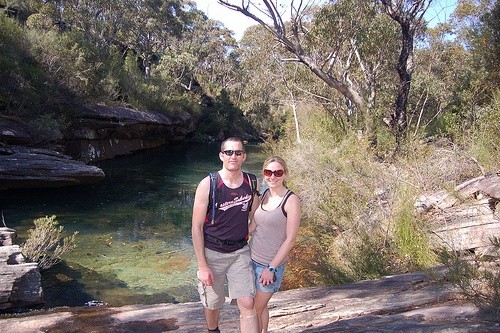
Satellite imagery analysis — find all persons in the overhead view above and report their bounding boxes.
[247,156,300,333]
[192,137,259,333]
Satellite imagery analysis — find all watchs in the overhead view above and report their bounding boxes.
[267,264,278,273]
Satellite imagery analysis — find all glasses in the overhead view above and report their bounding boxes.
[222,150,244,156]
[264,169,286,177]
